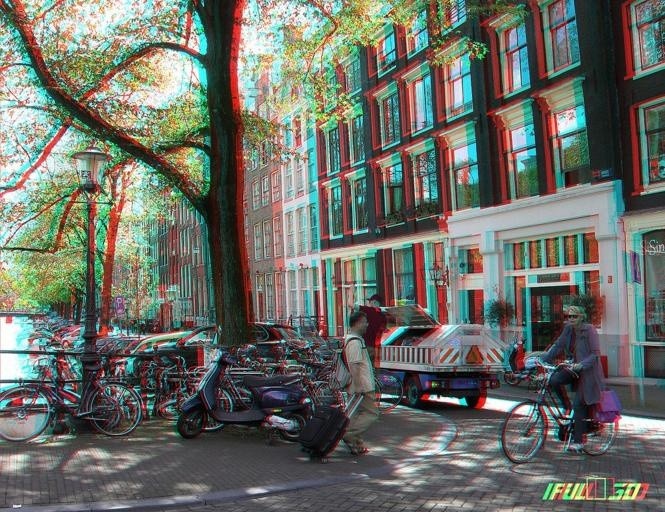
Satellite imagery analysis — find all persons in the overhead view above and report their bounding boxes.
[338,311,381,457]
[520,304,605,453]
[357,294,386,366]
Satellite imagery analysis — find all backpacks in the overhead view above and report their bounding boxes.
[329,336,362,390]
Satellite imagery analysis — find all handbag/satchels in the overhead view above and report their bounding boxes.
[595,390,620,423]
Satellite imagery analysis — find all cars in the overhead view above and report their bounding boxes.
[48,316,309,391]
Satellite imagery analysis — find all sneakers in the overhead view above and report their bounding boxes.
[563,407,574,419]
[570,442,584,450]
[344,437,366,457]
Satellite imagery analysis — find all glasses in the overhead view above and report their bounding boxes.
[567,314,583,319]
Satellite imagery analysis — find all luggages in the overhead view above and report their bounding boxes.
[298,392,365,463]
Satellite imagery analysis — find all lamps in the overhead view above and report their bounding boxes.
[430,262,450,287]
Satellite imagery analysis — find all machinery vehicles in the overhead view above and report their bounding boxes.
[375,304,511,408]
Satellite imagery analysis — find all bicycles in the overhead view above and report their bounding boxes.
[0,354,145,442]
[501,356,618,464]
[157,343,403,441]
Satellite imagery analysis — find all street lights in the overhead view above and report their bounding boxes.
[71,146,111,433]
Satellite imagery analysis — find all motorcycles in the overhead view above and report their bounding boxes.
[504,339,544,385]
